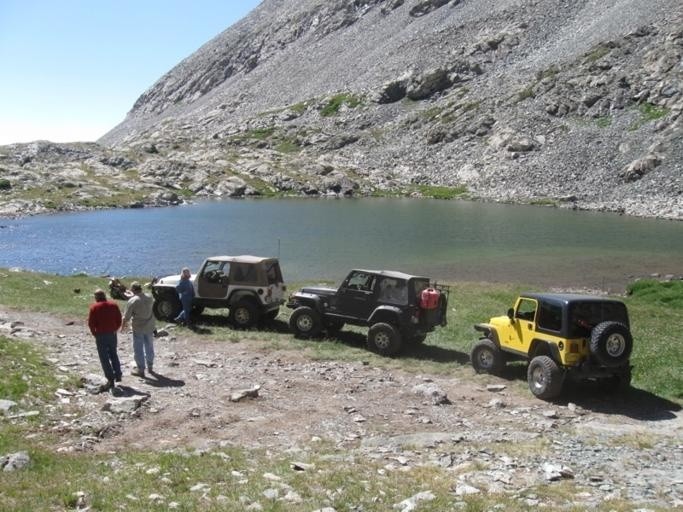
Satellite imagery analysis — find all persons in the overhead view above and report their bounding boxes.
[173,267,198,331]
[108,276,159,301]
[120,280,155,377]
[88,289,123,391]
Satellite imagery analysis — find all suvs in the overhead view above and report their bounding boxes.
[146,254,288,330]
[284,266,453,357]
[467,290,637,404]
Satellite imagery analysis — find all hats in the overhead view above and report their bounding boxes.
[94,288,106,300]
[128,280,141,292]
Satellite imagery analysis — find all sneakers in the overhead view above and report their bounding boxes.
[107,318,188,382]
